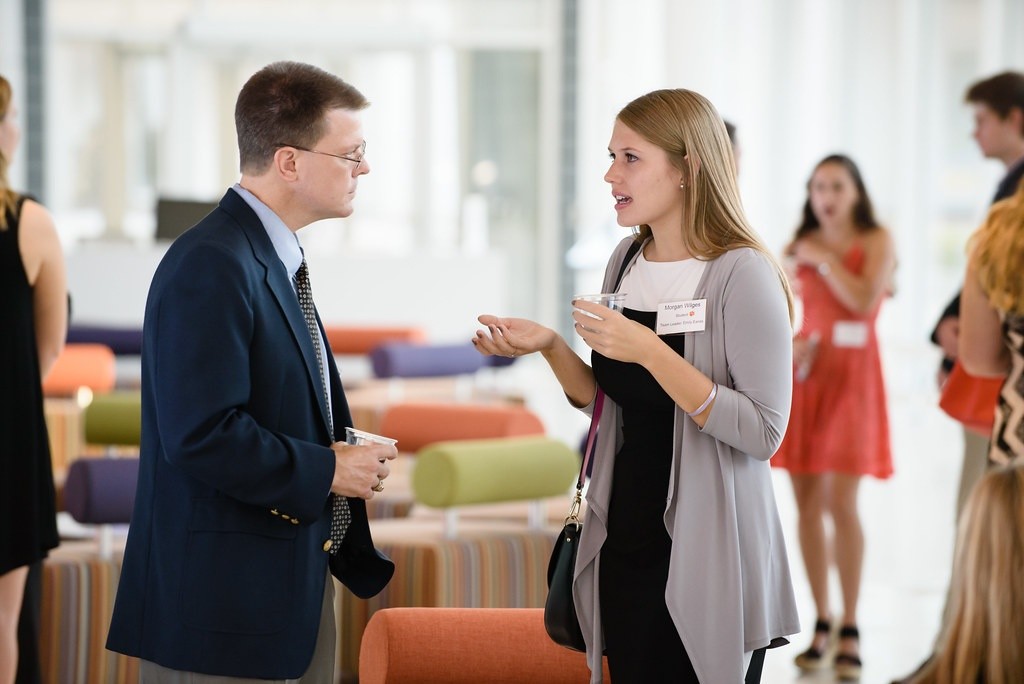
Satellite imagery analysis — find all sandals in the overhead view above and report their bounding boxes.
[796,619,837,670]
[836,625,861,679]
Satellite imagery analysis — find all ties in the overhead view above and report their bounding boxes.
[295,247,352,555]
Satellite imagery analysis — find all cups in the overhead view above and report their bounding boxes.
[570,292,627,333]
[345,426,399,465]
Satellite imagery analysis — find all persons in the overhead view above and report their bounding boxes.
[105,60,398,684]
[472,89,800,684]
[771,154,897,679]
[891,71,1024,684]
[0,74,67,684]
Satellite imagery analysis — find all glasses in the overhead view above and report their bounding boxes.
[270,139,366,169]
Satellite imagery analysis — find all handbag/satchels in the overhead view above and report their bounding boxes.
[541,522,607,657]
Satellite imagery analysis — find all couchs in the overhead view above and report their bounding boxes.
[314,325,610,684]
[37,322,170,684]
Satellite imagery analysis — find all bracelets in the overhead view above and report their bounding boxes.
[687,383,716,417]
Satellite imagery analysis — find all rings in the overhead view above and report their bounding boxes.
[509,348,517,356]
[372,480,384,492]
